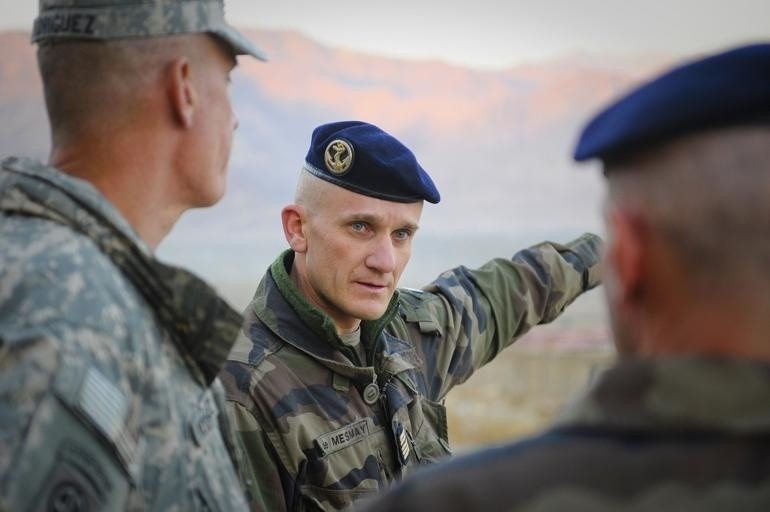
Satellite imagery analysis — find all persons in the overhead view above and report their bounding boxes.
[218,120,607,511]
[349,43,769,511]
[1,0,279,511]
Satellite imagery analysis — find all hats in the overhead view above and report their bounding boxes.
[569,39,770,176]
[29,0,270,65]
[302,119,442,207]
